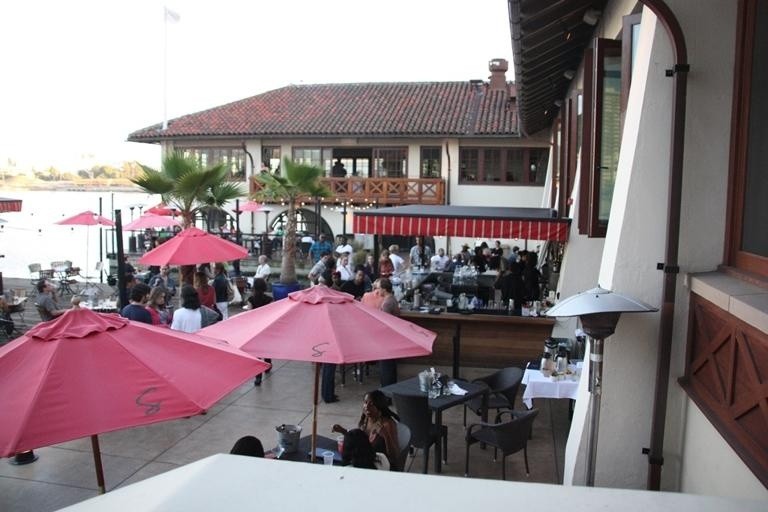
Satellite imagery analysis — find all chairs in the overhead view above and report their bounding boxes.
[139,232,312,265]
[464,367,539,480]
[392,392,448,473]
[0,260,80,342]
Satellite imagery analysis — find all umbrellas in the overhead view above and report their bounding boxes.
[0,296,271,492]
[232,202,267,236]
[189,283,438,461]
[144,202,183,218]
[56,211,115,281]
[122,213,180,252]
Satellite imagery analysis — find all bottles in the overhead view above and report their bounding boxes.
[281,424,285,432]
[412,289,515,312]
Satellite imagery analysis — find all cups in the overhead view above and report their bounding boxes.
[336,435,344,452]
[323,451,334,466]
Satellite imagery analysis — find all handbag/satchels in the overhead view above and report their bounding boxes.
[210,306,223,321]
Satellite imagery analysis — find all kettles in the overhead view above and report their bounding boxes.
[541,337,568,376]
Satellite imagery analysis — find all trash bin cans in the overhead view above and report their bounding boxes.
[271,282,301,300]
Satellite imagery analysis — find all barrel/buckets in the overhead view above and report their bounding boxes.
[419,371,441,391]
[3,288,16,303]
[274,424,303,455]
[273,281,304,300]
[230,278,247,293]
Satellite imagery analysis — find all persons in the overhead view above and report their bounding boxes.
[428,241,503,273]
[125,262,176,325]
[230,436,266,457]
[143,225,180,250]
[0,295,17,336]
[358,390,401,456]
[410,237,431,268]
[495,247,540,314]
[332,158,407,207]
[248,255,272,385]
[38,277,70,320]
[330,426,393,471]
[322,286,339,403]
[180,261,230,321]
[300,231,405,298]
[375,278,400,386]
[256,225,276,247]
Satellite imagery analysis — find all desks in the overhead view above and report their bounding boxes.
[265,434,350,465]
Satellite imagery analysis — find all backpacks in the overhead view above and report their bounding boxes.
[222,272,234,302]
